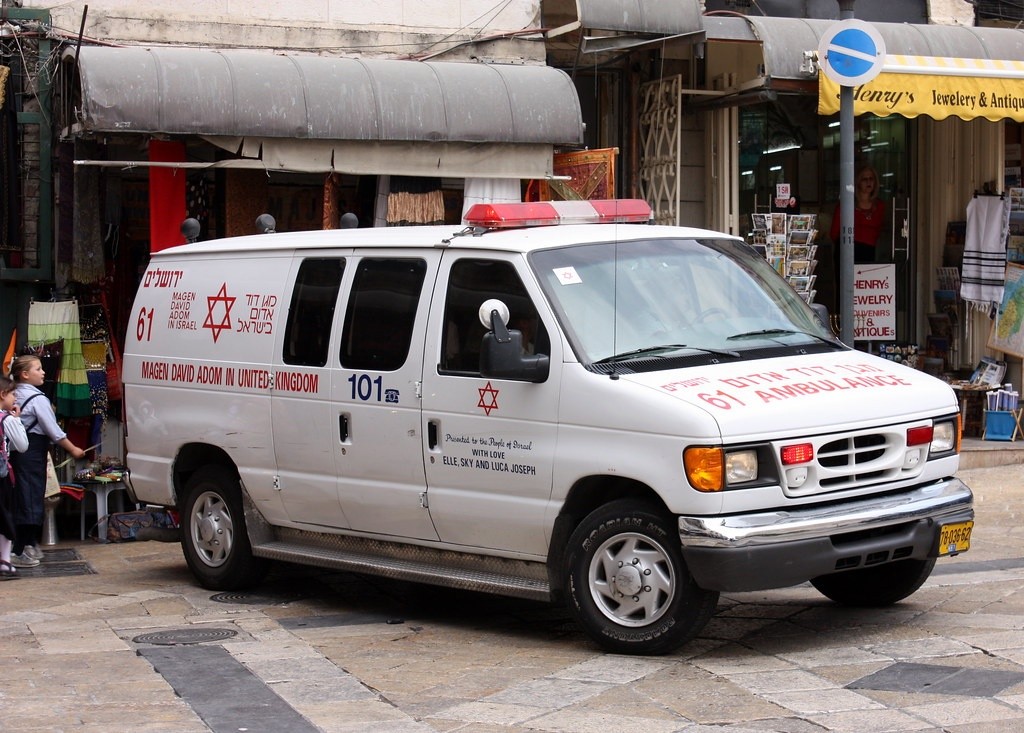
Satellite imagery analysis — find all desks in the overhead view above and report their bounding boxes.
[949,380,1001,432]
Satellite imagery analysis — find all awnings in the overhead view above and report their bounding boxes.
[804,50,1024,124]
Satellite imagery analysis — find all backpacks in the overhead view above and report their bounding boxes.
[0,413,17,488]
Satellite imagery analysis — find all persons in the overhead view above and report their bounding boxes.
[0,356,85,580]
[830,164,888,263]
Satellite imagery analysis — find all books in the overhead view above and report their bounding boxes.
[749,213,817,307]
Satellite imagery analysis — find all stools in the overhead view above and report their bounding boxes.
[81,482,141,542]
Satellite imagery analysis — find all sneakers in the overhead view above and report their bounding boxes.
[23,546,44,560]
[10,553,40,567]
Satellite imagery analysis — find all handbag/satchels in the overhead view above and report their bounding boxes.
[89,508,180,544]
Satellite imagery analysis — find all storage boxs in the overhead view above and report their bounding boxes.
[980,408,1024,442]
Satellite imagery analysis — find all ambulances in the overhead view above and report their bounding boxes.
[121,199,974,655]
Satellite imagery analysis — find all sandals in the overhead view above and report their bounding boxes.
[0,560,21,580]
